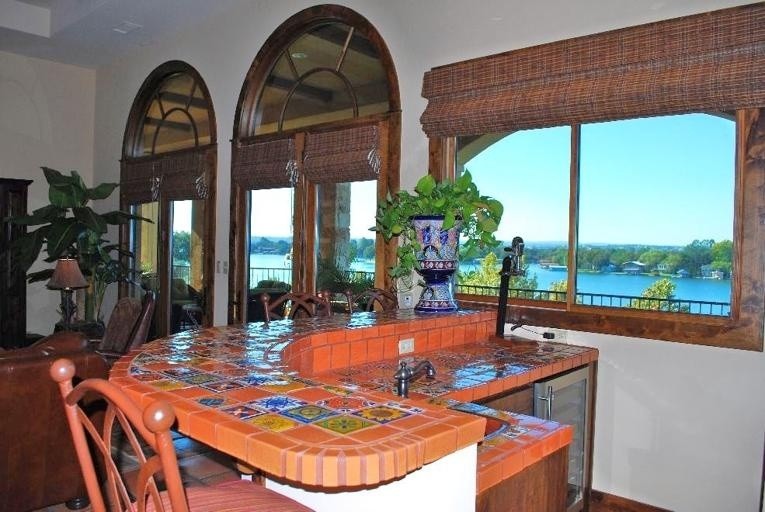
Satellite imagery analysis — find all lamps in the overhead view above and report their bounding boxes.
[45,258,90,329]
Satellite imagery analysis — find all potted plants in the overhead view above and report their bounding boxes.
[368,169,505,315]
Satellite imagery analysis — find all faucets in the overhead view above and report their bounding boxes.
[502,236,527,278]
[395,358,437,398]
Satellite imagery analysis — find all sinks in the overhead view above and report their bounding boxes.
[448,405,508,443]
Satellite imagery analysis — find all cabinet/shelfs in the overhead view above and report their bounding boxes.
[534,363,597,511]
